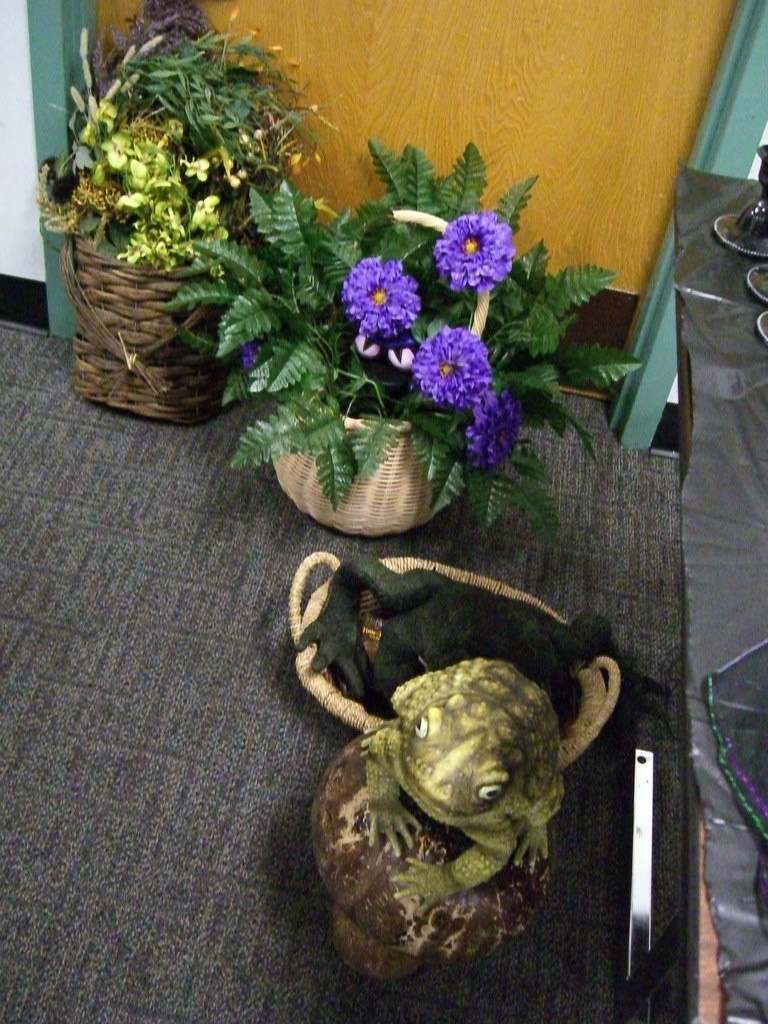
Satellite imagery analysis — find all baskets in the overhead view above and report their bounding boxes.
[287,553,624,774]
[266,213,491,540]
[61,212,228,425]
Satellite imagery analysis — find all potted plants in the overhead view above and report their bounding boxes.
[54,207,230,425]
[255,382,493,539]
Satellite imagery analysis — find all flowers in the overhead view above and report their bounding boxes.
[39,5,299,278]
[178,140,633,532]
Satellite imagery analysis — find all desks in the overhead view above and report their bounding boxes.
[663,163,768,1019]
[0,316,695,1015]
